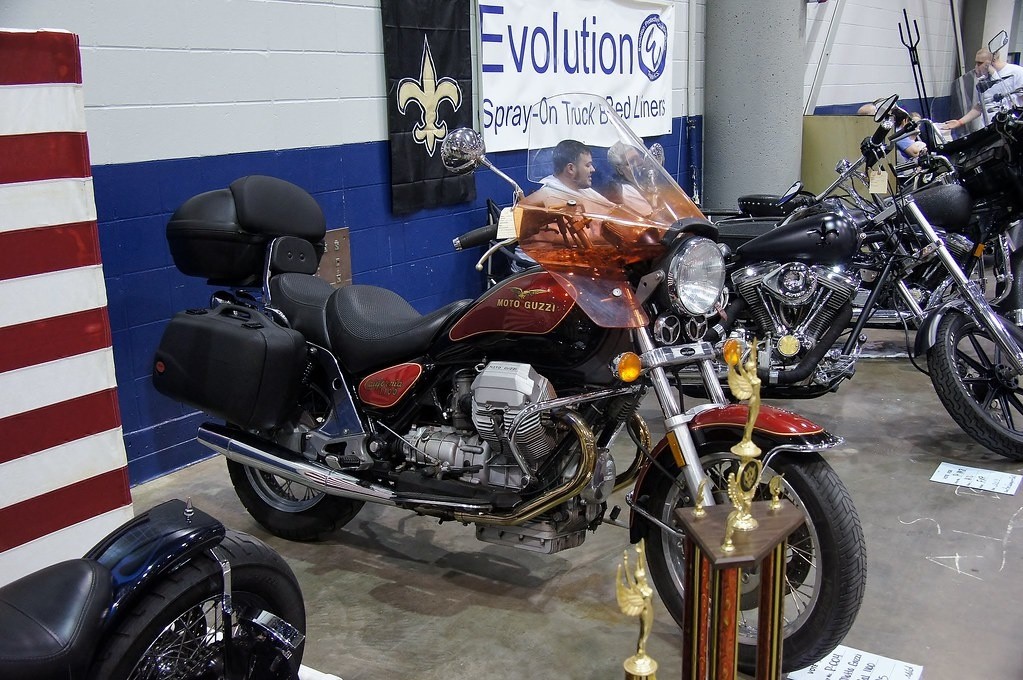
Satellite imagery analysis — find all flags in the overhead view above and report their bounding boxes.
[380,0,476,215]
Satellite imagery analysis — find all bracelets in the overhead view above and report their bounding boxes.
[958,120,963,127]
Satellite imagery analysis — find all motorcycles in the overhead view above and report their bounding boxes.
[487,123,1023,461]
[697,29,1023,325]
[0,499,305,680]
[153,93,866,675]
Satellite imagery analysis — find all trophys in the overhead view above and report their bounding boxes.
[617,335,804,680]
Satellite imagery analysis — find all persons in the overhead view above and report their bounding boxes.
[511,139,614,274]
[607,141,645,204]
[858,104,928,174]
[941,47,1023,131]
